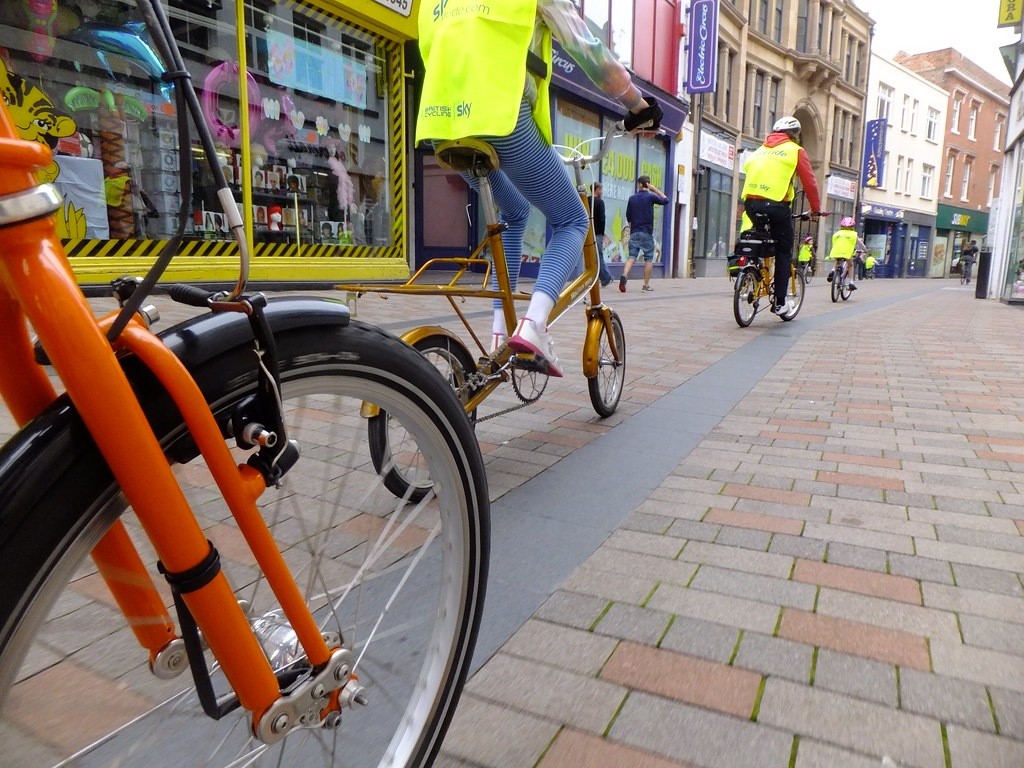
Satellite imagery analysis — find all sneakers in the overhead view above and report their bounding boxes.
[508,318,565,377]
[771,300,796,315]
[489,333,508,353]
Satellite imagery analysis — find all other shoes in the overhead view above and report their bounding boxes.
[849,283,857,290]
[601,276,615,288]
[640,285,654,293]
[827,270,835,282]
[619,275,627,292]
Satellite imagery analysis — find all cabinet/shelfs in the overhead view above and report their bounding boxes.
[228,188,315,247]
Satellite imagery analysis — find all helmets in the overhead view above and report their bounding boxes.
[773,116,801,131]
[971,240,977,243]
[805,236,813,242]
[840,217,855,227]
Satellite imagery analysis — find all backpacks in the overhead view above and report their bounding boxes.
[963,242,973,253]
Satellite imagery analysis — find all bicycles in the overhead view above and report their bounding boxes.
[332,121,662,504]
[734,211,852,328]
[960,259,977,285]
[1,0,493,768]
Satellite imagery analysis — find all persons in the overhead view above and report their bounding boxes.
[269,173,279,189]
[711,237,726,257]
[298,211,305,225]
[619,175,670,293]
[321,223,336,240]
[797,236,818,275]
[286,176,301,193]
[958,240,979,283]
[256,207,265,223]
[741,116,821,317]
[267,205,283,232]
[414,0,663,377]
[255,171,263,188]
[827,216,879,291]
[739,210,753,233]
[585,182,616,287]
[320,135,354,209]
[214,214,224,232]
[205,213,214,231]
[336,223,343,238]
